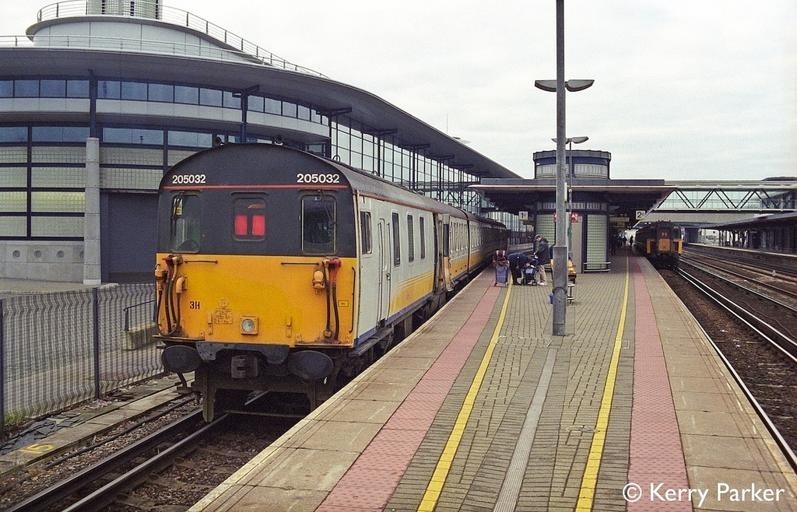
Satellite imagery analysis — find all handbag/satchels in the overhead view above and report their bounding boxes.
[493,249,509,265]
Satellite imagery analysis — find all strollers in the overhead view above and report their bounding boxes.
[522,250,537,287]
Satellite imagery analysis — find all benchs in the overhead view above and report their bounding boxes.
[548,258,575,305]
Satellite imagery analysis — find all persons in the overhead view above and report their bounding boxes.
[508,253,532,285]
[609,234,633,256]
[534,233,550,285]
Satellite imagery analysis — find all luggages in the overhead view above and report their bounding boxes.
[494,264,509,288]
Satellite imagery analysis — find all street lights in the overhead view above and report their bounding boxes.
[549,135,590,259]
[535,76,594,338]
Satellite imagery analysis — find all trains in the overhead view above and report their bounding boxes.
[632,219,682,266]
[151,139,513,424]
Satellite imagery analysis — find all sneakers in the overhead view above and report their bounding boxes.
[537,281,548,286]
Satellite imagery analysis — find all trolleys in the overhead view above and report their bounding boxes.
[491,249,509,288]
